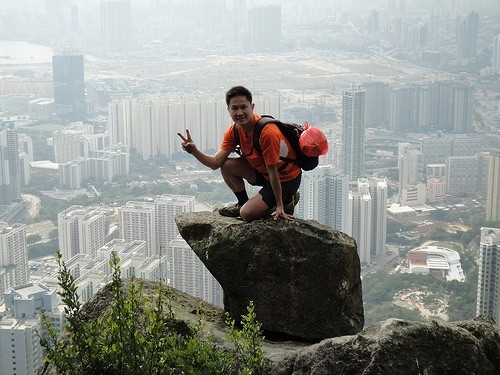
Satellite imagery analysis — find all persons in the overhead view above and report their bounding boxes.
[177,85,302,222]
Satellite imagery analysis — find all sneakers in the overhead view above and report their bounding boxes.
[218,201,245,218]
[283,191,301,218]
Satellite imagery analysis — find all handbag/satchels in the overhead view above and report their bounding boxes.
[298,120,329,157]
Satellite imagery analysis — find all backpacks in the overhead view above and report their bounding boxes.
[232,113,319,171]
